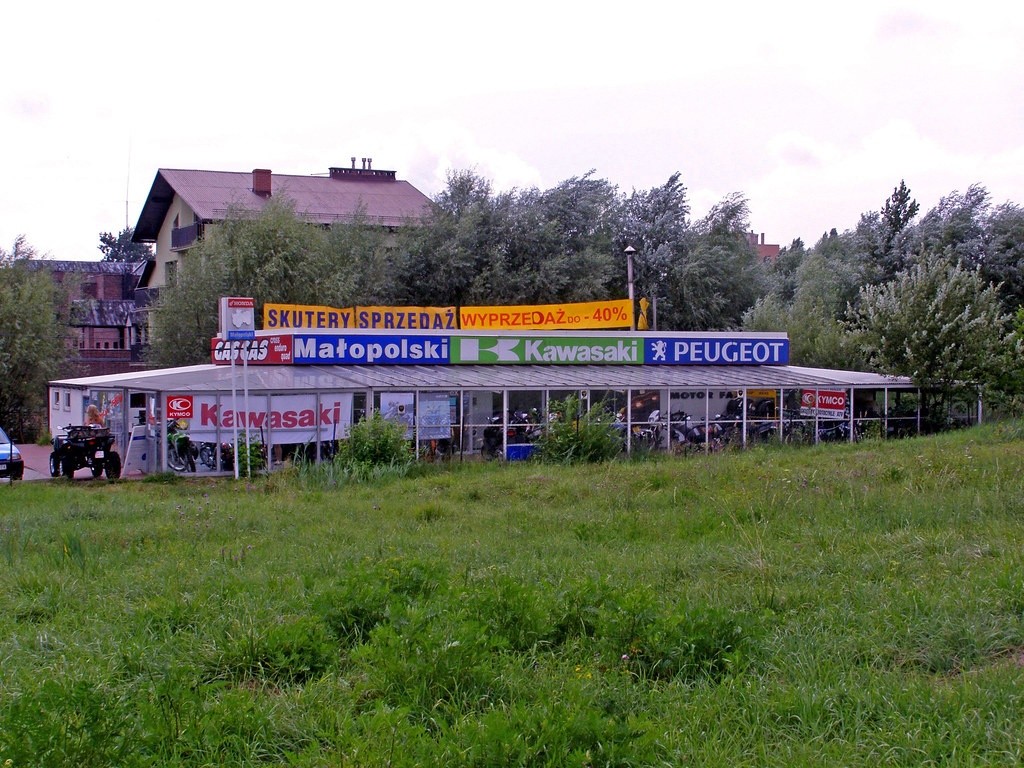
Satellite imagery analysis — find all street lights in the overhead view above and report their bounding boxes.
[623,245,637,331]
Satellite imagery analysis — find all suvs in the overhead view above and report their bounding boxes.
[49,422,122,484]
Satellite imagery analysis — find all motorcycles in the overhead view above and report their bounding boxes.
[155,415,200,473]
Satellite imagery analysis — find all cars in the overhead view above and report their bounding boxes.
[0,426,24,480]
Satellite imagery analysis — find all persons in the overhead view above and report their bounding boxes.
[83,404,105,479]
[274,443,306,464]
[435,427,458,463]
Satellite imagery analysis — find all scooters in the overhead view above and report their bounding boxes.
[199,397,900,476]
